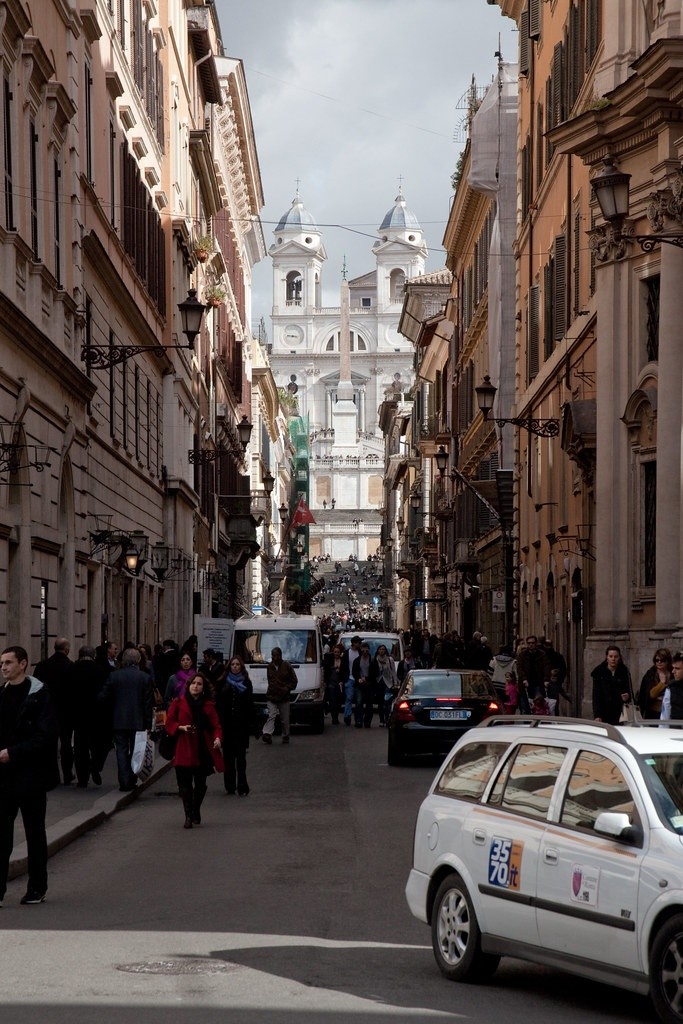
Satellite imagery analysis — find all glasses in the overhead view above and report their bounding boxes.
[361,649,369,653]
[655,658,667,662]
[527,641,535,644]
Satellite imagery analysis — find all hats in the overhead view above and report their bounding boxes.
[404,648,412,652]
[351,636,365,643]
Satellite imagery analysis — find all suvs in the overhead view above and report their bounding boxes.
[406,715,683,1024]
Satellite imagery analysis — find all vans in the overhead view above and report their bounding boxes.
[335,629,405,707]
[230,614,324,732]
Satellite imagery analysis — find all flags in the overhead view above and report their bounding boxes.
[291,499,316,526]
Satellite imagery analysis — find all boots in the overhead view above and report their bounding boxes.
[192,784,208,825]
[179,784,193,829]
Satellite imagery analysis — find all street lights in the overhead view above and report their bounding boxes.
[475,375,560,440]
[410,489,453,522]
[281,542,304,560]
[81,288,206,369]
[396,516,414,540]
[434,446,461,482]
[386,534,399,552]
[590,153,683,253]
[264,503,288,527]
[188,414,254,465]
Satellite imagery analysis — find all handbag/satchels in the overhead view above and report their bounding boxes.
[383,686,399,705]
[618,703,642,722]
[131,728,156,782]
[158,725,181,761]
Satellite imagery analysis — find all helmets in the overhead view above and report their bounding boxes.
[472,631,483,641]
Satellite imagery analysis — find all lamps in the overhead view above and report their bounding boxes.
[386,534,393,550]
[434,446,480,481]
[187,414,253,465]
[311,566,316,576]
[280,542,302,559]
[589,152,683,252]
[218,471,275,498]
[409,491,437,515]
[79,288,207,370]
[91,528,137,575]
[120,530,149,576]
[396,517,414,537]
[142,541,171,583]
[474,376,560,438]
[261,503,289,527]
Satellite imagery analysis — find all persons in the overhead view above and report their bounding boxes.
[214,656,254,797]
[261,647,298,744]
[398,624,493,691]
[312,547,384,654]
[489,636,573,716]
[332,498,336,509]
[639,648,683,728]
[324,636,397,728]
[323,500,327,508]
[309,427,380,465]
[33,638,154,792]
[0,646,48,904]
[164,672,222,829]
[590,645,634,725]
[139,634,226,798]
[353,518,363,526]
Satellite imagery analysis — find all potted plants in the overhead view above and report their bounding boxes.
[209,288,227,307]
[195,236,211,261]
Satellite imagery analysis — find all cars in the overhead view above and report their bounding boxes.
[388,669,508,767]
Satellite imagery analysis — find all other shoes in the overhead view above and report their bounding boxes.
[63,774,76,785]
[329,715,391,731]
[75,780,87,789]
[262,733,273,745]
[282,736,290,746]
[88,763,102,785]
[19,884,49,904]
